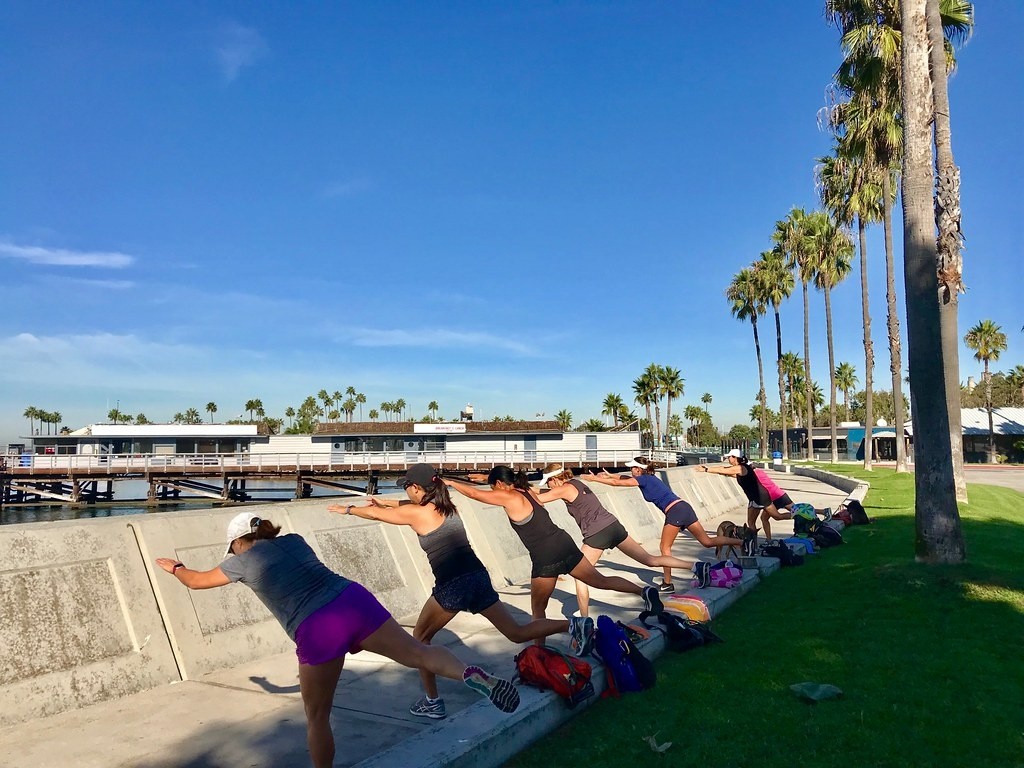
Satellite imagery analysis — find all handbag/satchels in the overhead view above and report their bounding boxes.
[789,502,845,547]
[832,499,869,527]
[759,538,804,570]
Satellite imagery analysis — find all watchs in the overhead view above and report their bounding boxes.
[347,505,356,515]
[173,563,185,575]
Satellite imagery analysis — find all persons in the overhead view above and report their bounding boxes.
[536,463,711,618]
[156,512,520,768]
[327,462,594,719]
[694,449,832,556]
[580,456,755,593]
[441,465,664,646]
[242,446,248,457]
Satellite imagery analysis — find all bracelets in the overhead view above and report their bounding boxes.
[705,468,708,472]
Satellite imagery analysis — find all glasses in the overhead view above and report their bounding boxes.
[402,482,412,491]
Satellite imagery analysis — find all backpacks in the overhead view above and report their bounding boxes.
[640,609,723,654]
[509,643,596,709]
[689,559,744,589]
[591,614,658,700]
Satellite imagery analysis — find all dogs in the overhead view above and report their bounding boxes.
[715,521,762,563]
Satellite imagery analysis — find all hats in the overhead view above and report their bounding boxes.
[222,512,264,560]
[396,463,437,486]
[724,448,744,458]
[625,459,648,469]
[538,467,564,486]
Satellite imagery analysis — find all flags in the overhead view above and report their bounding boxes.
[461,411,467,418]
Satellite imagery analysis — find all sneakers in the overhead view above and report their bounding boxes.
[568,615,594,657]
[824,507,832,522]
[642,585,664,613]
[692,560,711,589]
[742,539,755,557]
[655,578,675,594]
[409,695,447,719]
[462,665,520,714]
